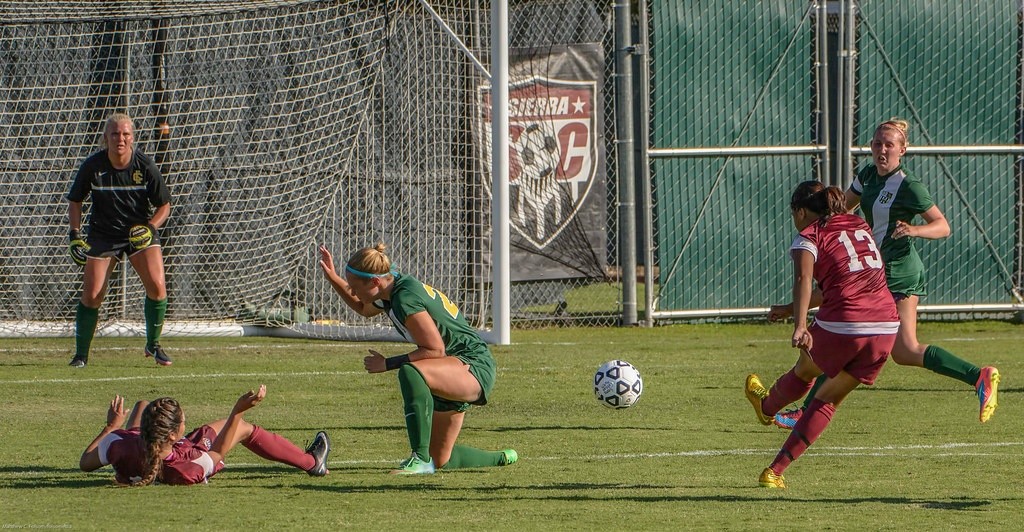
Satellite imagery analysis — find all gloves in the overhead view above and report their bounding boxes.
[130,224,156,251]
[69,228,91,265]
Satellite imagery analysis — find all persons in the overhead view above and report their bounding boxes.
[316,243,519,476]
[744,178,901,490]
[67,114,174,369]
[79,381,330,487]
[773,120,999,432]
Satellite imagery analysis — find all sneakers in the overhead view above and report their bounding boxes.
[69,355,88,367]
[145,341,171,365]
[488,449,518,464]
[975,366,999,423]
[760,467,786,489]
[305,431,330,476]
[774,409,805,430]
[390,453,435,474]
[745,373,774,427]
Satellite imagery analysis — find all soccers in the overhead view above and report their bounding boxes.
[590,357,646,413]
